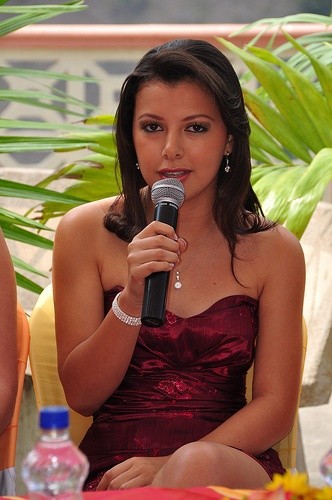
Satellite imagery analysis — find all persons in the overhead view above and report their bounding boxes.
[0,223,19,434]
[51,38,307,492]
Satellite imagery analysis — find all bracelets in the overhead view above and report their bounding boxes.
[112,292,142,327]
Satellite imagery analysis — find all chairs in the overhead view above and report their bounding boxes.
[31,282,311,485]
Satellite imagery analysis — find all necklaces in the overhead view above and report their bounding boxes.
[147,214,213,289]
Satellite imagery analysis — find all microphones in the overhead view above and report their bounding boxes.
[141,178,185,326]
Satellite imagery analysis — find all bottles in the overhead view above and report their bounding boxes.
[20,407,90,500]
